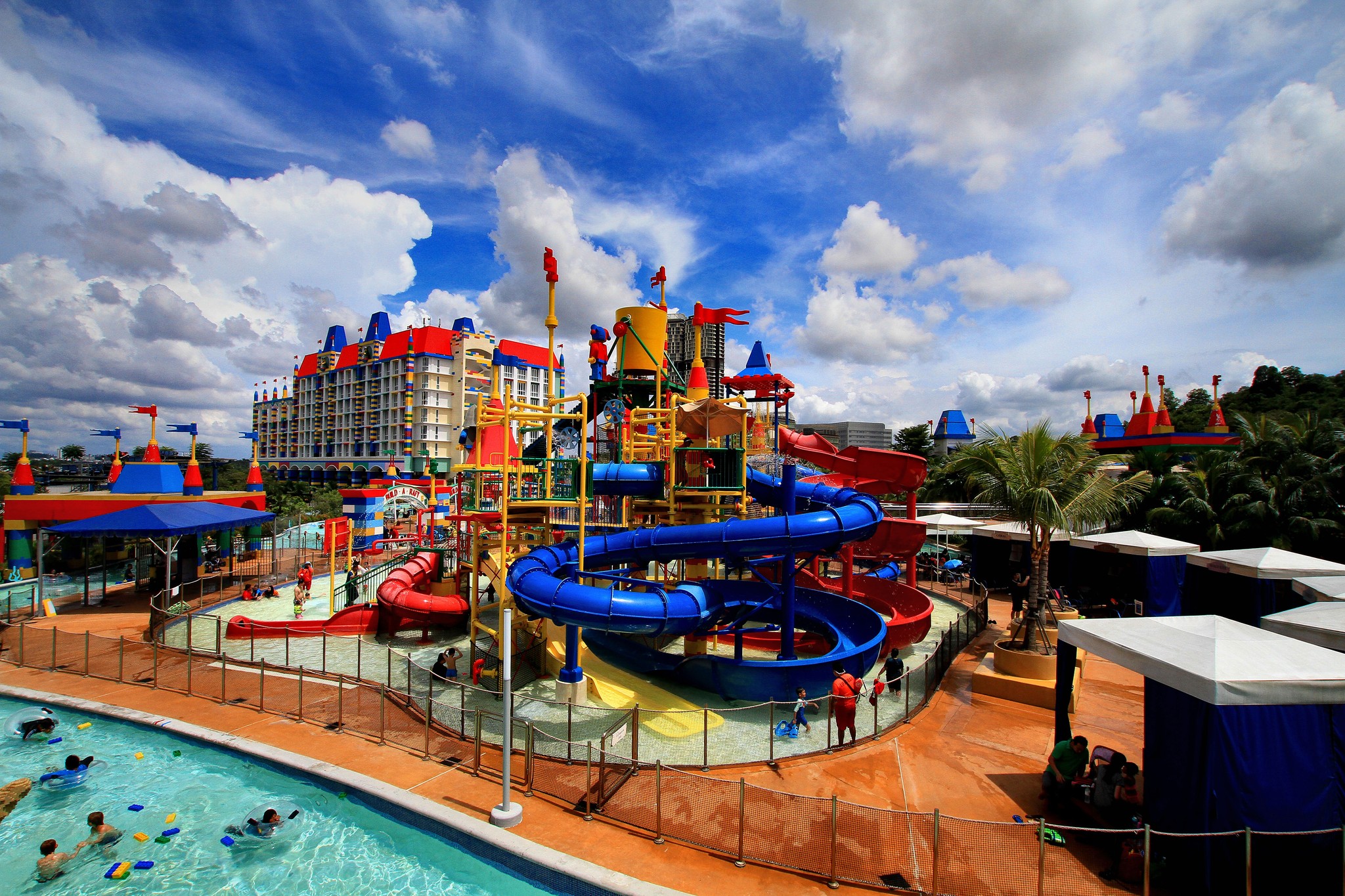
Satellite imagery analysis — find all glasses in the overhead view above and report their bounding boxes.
[685,439,692,442]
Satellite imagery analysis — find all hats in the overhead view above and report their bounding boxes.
[590,324,611,342]
[683,437,694,444]
[833,661,845,675]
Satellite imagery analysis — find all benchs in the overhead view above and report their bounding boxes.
[1072,793,1115,829]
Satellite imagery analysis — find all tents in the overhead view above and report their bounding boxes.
[912,514,987,583]
[1054,614,1345,896]
[968,516,1344,654]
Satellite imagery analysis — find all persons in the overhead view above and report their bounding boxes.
[1121,762,1140,805]
[13,717,55,741]
[877,648,904,704]
[33,839,83,883]
[1093,752,1129,830]
[443,648,463,685]
[75,812,124,851]
[792,687,819,739]
[830,662,857,751]
[432,653,458,682]
[567,368,770,564]
[225,808,284,841]
[916,549,1031,629]
[40,459,551,619]
[30,754,94,791]
[1042,735,1089,811]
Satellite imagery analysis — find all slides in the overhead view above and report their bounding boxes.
[224,422,935,740]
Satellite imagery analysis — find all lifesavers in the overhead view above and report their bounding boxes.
[2,706,60,739]
[241,800,305,842]
[37,574,72,585]
[43,585,79,595]
[42,760,109,791]
[76,575,97,582]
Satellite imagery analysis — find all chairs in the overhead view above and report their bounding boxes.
[1054,588,1089,612]
